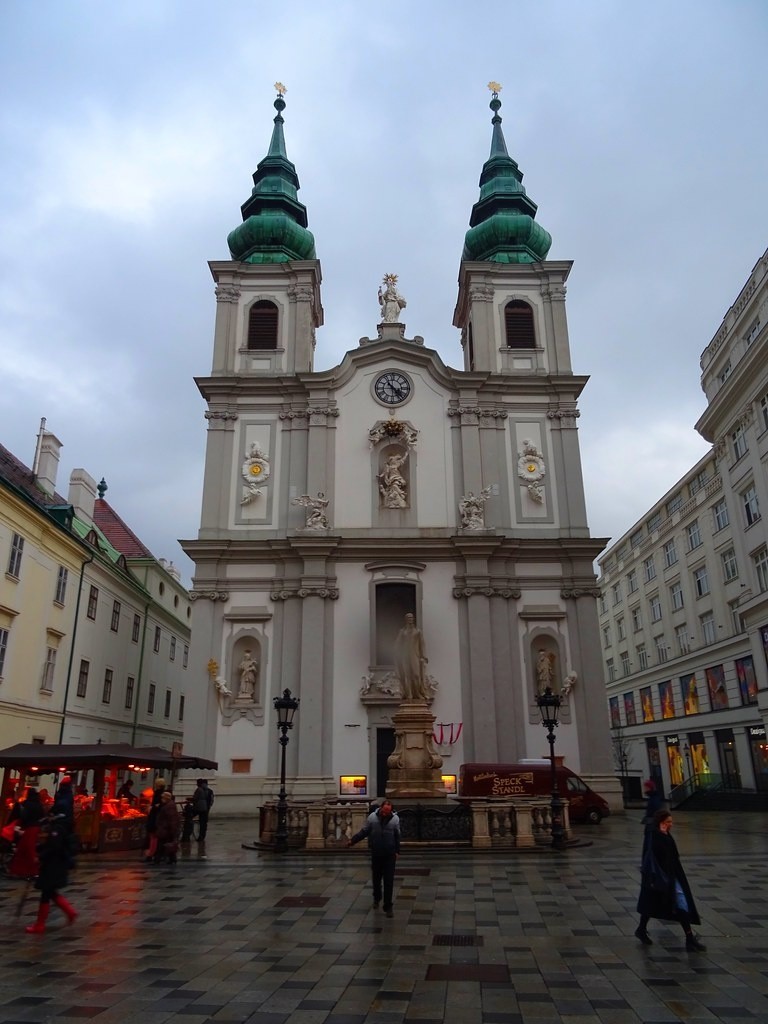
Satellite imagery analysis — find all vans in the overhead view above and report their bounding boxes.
[458,759,610,826]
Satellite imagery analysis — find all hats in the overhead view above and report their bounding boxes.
[655,811,671,825]
[161,792,172,800]
[645,780,655,789]
[155,778,165,786]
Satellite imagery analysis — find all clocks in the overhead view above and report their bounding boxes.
[375,373,410,404]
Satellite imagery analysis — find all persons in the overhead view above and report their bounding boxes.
[359,670,378,695]
[537,649,554,696]
[0,775,214,934]
[378,273,407,322]
[237,648,257,697]
[640,780,665,862]
[345,800,402,917]
[392,613,429,700]
[561,671,577,695]
[634,808,709,951]
[215,677,232,696]
[239,450,544,531]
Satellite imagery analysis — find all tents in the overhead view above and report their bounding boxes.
[0,743,218,801]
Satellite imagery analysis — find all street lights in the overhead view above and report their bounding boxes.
[536,686,567,849]
[271,688,298,854]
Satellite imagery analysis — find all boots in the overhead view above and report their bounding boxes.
[24,903,49,934]
[52,893,79,926]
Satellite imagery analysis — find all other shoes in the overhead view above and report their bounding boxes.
[686,935,708,953]
[387,908,394,918]
[373,901,379,910]
[634,926,652,946]
